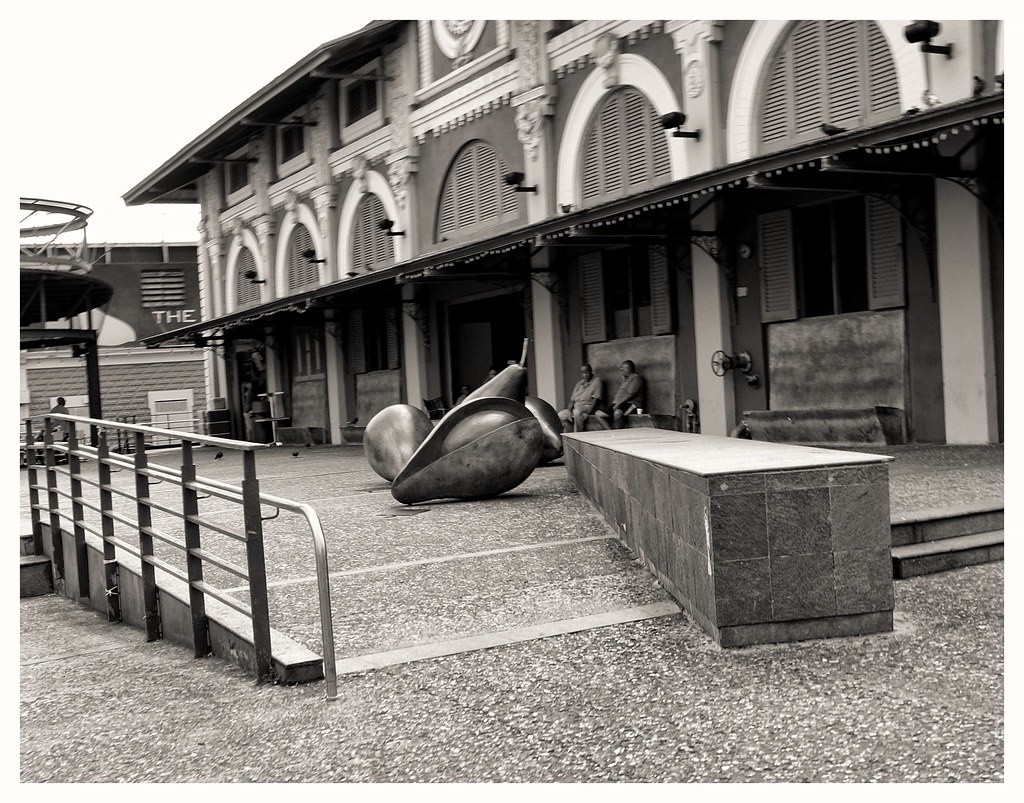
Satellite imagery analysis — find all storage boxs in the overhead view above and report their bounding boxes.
[197,398,231,446]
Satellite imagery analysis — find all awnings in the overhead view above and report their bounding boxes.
[138,89,1004,349]
[122,20,410,204]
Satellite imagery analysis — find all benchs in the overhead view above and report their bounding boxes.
[584,413,677,431]
[742,405,905,447]
[340,426,368,446]
[277,427,327,445]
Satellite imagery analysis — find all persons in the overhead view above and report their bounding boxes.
[594,360,645,431]
[558,362,602,433]
[453,358,518,405]
[36,395,71,463]
[243,381,267,441]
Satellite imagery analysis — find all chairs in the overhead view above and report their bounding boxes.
[422,396,450,421]
